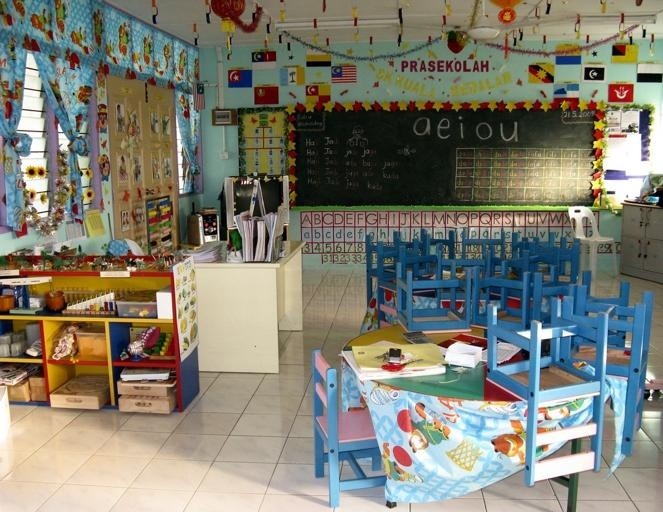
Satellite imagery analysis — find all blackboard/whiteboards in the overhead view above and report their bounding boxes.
[284,100,607,211]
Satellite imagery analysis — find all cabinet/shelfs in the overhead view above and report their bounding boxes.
[187,209,220,246]
[621,202,663,284]
[0,255,199,412]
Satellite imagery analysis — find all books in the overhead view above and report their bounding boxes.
[340,339,446,381]
[119,368,169,381]
[193,211,279,262]
[83,209,107,238]
[353,344,446,372]
[0,362,39,386]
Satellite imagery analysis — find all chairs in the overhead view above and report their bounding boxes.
[359,228,630,348]
[312,349,387,508]
[484,298,603,488]
[564,285,654,457]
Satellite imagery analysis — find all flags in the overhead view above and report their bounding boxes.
[225,48,359,105]
[528,40,662,104]
[192,82,206,111]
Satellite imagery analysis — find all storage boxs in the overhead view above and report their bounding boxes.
[74,325,107,357]
[115,289,159,318]
[8,376,48,403]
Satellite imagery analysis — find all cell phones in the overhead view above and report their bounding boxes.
[388,348,401,364]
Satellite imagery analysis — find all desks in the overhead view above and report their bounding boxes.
[195,237,306,373]
[343,316,632,511]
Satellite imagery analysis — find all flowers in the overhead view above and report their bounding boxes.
[23,143,95,237]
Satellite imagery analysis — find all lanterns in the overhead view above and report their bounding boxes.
[209,1,245,34]
[488,0,523,10]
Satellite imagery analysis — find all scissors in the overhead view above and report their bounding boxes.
[381,359,422,371]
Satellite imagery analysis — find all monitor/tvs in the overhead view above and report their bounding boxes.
[218,174,289,229]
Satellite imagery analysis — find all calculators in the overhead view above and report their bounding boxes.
[401,331,435,344]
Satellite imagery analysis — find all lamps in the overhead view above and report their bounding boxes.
[576,14,656,25]
[467,27,500,42]
[275,18,404,35]
